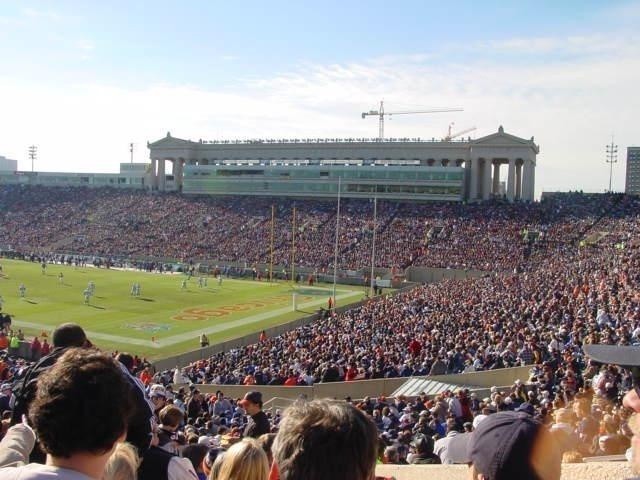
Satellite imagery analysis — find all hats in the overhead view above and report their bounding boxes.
[464,411,560,480]
[583,343,640,366]
[53,323,92,348]
[149,384,167,396]
[239,392,262,405]
[410,437,434,452]
